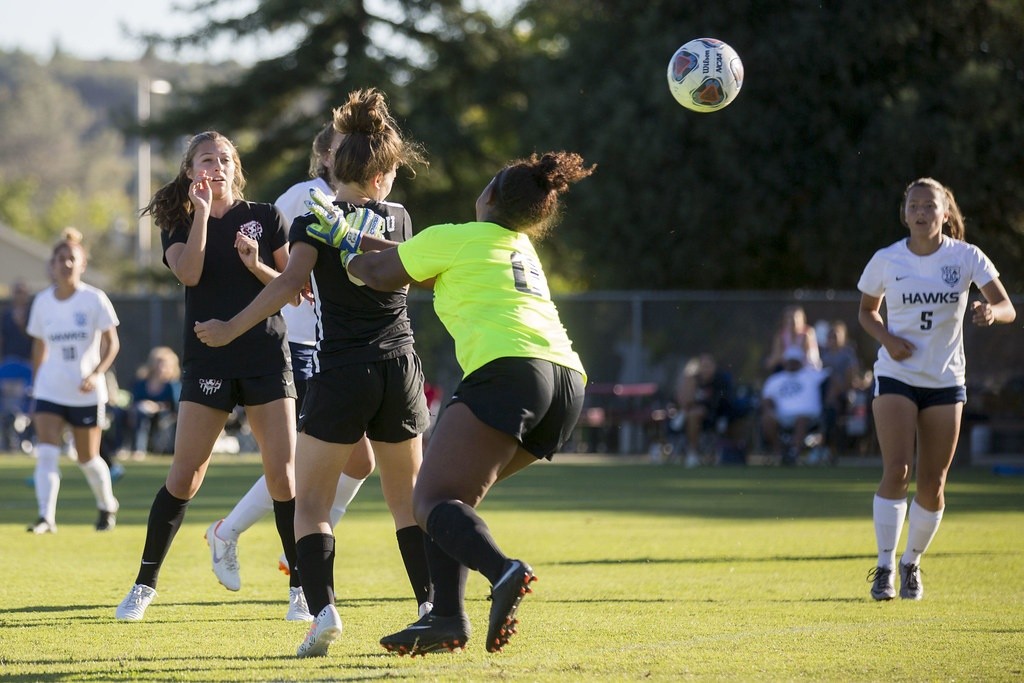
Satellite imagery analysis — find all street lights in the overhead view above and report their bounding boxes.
[135,71,173,252]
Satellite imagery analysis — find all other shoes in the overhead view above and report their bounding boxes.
[27,519,57,534]
[96,511,115,531]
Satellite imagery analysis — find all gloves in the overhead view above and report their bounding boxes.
[339,206,384,286]
[303,187,364,253]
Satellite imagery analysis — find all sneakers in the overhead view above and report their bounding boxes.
[418,601,433,618]
[867,566,896,600]
[899,555,924,599]
[286,586,315,622]
[380,611,469,656]
[204,519,240,592]
[115,583,159,621]
[296,604,343,658]
[278,553,290,575]
[485,559,537,652]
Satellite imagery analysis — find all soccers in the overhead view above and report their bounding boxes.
[666,37,745,114]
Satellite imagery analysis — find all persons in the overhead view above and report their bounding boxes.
[112,131,313,626]
[856,176,1016,600]
[190,88,464,655]
[301,153,590,654]
[25,228,121,535]
[204,125,376,614]
[681,305,1024,464]
[0,346,261,451]
